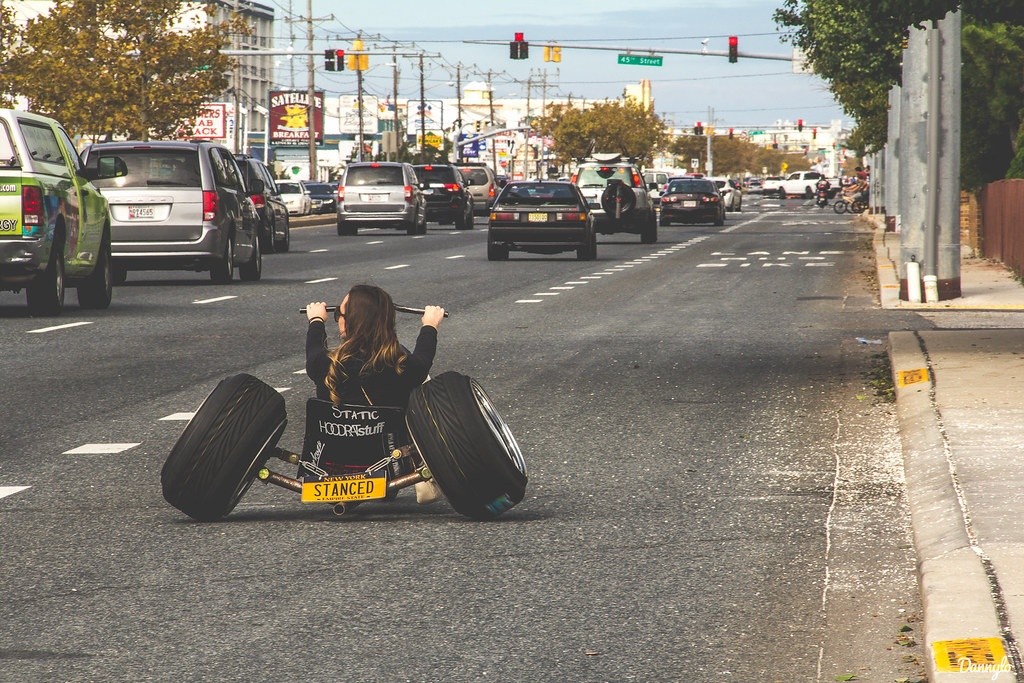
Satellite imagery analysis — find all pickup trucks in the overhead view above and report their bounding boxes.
[778,171,841,198]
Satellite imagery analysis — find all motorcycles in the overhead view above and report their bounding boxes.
[160,303,528,521]
[816,184,832,208]
[834,179,870,214]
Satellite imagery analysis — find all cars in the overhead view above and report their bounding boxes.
[732,176,784,195]
[0,108,112,316]
[274,179,339,215]
[487,174,597,261]
[642,173,743,226]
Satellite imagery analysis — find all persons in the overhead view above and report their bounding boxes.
[816,175,830,203]
[306,285,444,505]
[843,166,870,204]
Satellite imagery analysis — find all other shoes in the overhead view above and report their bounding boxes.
[414,481,442,504]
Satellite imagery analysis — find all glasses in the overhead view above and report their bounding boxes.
[334,306,344,323]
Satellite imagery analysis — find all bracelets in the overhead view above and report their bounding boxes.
[309,317,324,323]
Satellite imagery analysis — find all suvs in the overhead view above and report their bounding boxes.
[412,163,475,230]
[232,154,289,253]
[571,153,659,243]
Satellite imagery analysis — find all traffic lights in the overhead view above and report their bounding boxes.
[813,128,817,140]
[325,50,344,71]
[509,33,528,60]
[728,128,733,139]
[694,122,704,136]
[798,119,803,132]
[729,37,738,63]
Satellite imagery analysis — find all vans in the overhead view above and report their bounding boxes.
[337,161,434,236]
[457,166,501,215]
[79,140,265,285]
